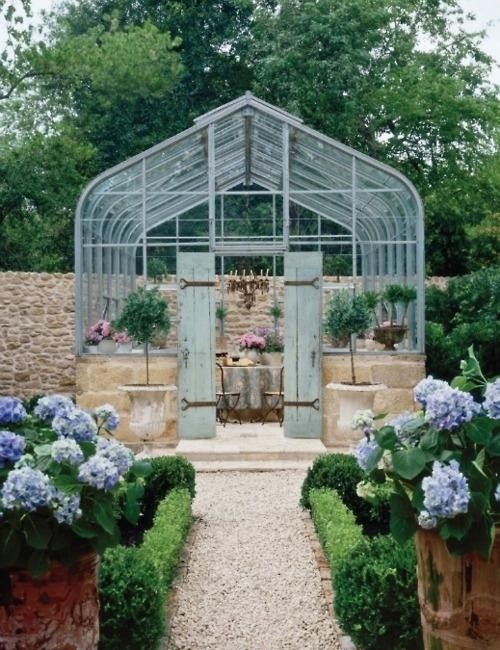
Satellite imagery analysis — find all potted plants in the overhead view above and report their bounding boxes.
[319,288,387,440]
[115,283,180,439]
[368,283,417,352]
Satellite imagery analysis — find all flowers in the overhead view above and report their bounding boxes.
[347,343,499,565]
[0,388,156,576]
[239,332,267,353]
[86,318,131,343]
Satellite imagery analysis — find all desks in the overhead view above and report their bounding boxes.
[217,364,285,411]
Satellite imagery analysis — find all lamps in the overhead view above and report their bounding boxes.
[226,265,273,310]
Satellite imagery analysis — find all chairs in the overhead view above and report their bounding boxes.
[260,365,287,428]
[214,360,242,428]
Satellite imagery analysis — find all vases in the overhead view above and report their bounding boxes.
[415,523,499,650]
[99,338,119,354]
[1,548,103,649]
[245,347,259,366]
